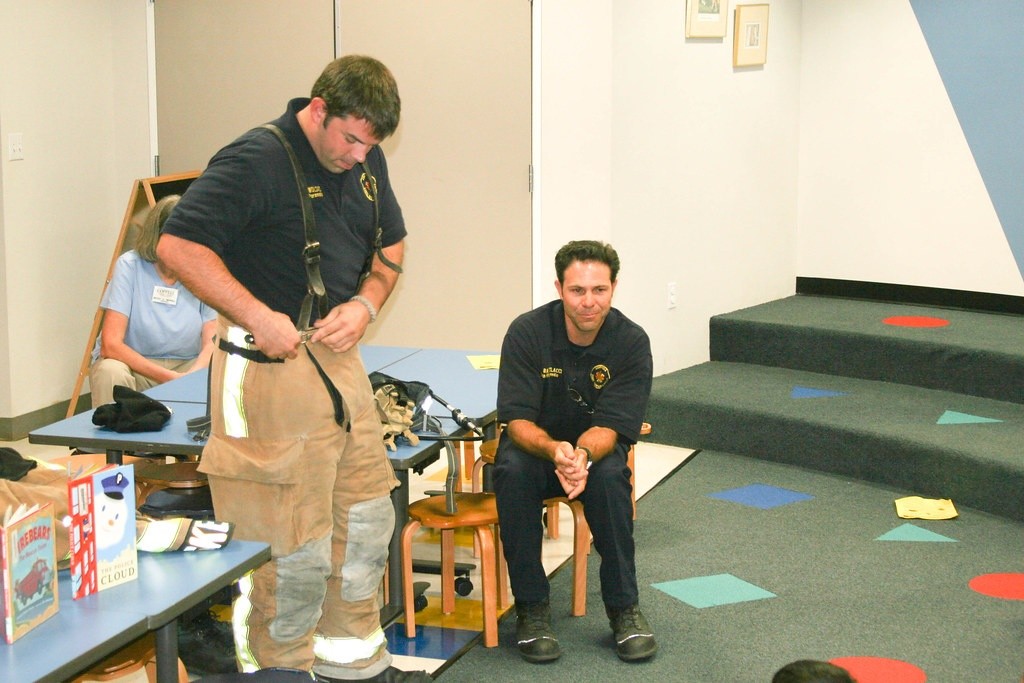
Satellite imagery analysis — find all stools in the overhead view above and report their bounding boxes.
[454,432,475,493]
[626,422,651,521]
[400,492,508,648]
[472,438,500,558]
[543,496,588,617]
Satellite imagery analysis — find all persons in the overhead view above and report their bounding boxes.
[86,193,217,408]
[491,240,657,661]
[155,55,432,683]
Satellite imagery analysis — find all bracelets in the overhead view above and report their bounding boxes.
[349,296,376,323]
[577,446,593,470]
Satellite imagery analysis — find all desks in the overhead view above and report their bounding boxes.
[28,345,501,630]
[0,540,271,683]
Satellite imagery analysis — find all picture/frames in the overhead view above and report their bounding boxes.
[733,4,770,66]
[686,0,729,38]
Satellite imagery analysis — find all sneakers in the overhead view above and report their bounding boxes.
[516,605,559,661]
[605,601,657,660]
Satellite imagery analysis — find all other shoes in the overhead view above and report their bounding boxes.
[314,666,432,683]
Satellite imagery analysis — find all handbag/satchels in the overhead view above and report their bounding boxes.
[368,371,458,512]
